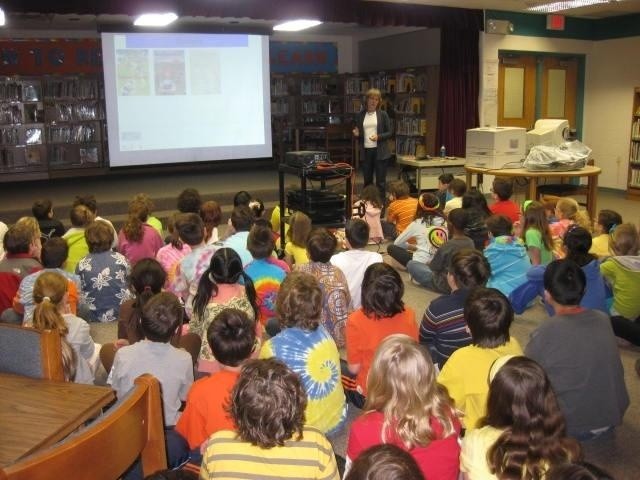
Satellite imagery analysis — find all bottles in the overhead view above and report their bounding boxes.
[440,144,446,162]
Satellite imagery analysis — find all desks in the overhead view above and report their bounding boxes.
[462,163,602,230]
[0,373,115,462]
[397,157,464,199]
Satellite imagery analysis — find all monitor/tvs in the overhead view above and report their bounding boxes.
[526,119,569,151]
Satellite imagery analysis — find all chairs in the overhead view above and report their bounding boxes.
[537,159,594,212]
[0,374,167,480]
[0,322,64,381]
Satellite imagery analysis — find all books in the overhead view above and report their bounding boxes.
[0,71,426,164]
[630,105,640,189]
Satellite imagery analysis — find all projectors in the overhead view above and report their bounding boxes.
[287,150,330,167]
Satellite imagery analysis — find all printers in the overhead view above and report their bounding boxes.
[466,127,527,171]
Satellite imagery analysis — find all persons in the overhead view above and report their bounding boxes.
[351,87,395,216]
[1,174,640,480]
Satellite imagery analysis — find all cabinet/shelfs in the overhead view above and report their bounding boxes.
[278,163,353,248]
[0,75,107,183]
[270,65,440,167]
[625,87,640,200]
